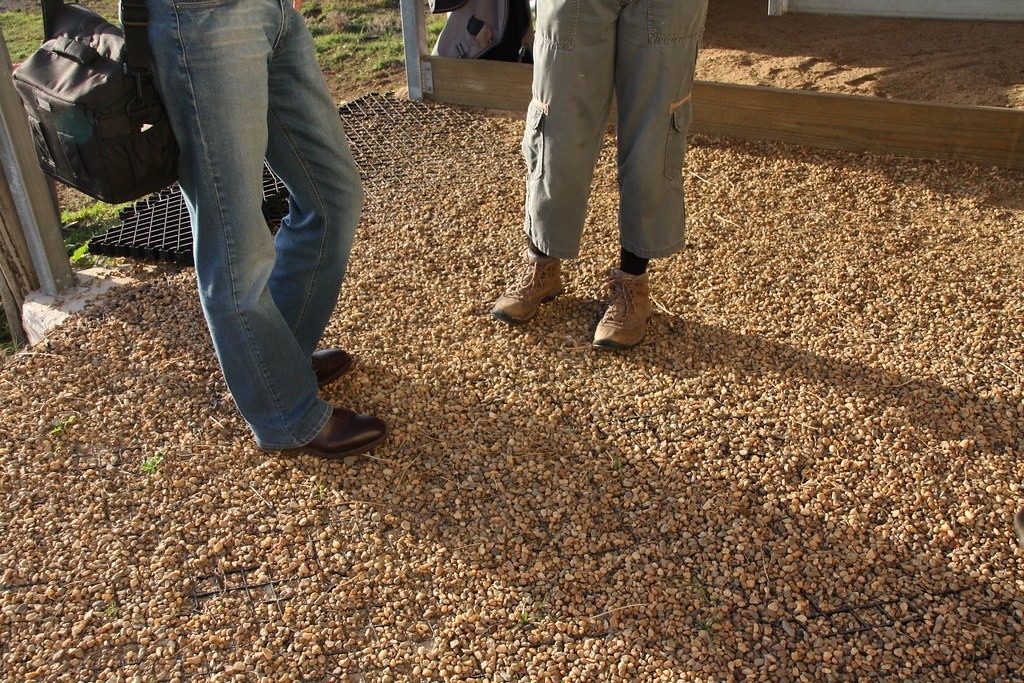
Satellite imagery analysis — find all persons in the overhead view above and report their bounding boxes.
[491,0,709,349]
[119,0,385,457]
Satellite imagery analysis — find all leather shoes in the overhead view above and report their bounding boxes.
[259,408,388,460]
[311,349,351,387]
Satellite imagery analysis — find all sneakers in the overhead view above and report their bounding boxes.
[492,249,562,325]
[593,268,652,348]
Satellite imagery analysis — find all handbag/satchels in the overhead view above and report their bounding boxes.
[12,3,183,205]
